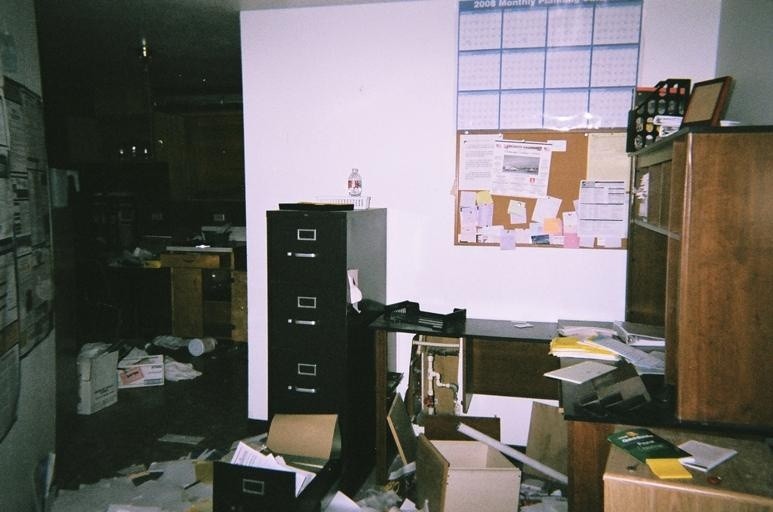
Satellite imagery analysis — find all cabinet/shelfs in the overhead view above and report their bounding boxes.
[626,126,773,425]
[213,209,387,512]
[163,238,247,342]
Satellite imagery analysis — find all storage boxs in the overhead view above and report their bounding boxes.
[75,350,119,414]
[414,434,523,511]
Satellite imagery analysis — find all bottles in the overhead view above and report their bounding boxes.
[347,167,363,208]
[188,337,219,357]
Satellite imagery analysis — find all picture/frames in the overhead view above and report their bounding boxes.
[684,76,732,126]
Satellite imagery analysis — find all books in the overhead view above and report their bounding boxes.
[543,319,665,385]
[605,427,738,480]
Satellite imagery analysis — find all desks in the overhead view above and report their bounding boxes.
[603,425,773,512]
[375,314,557,487]
